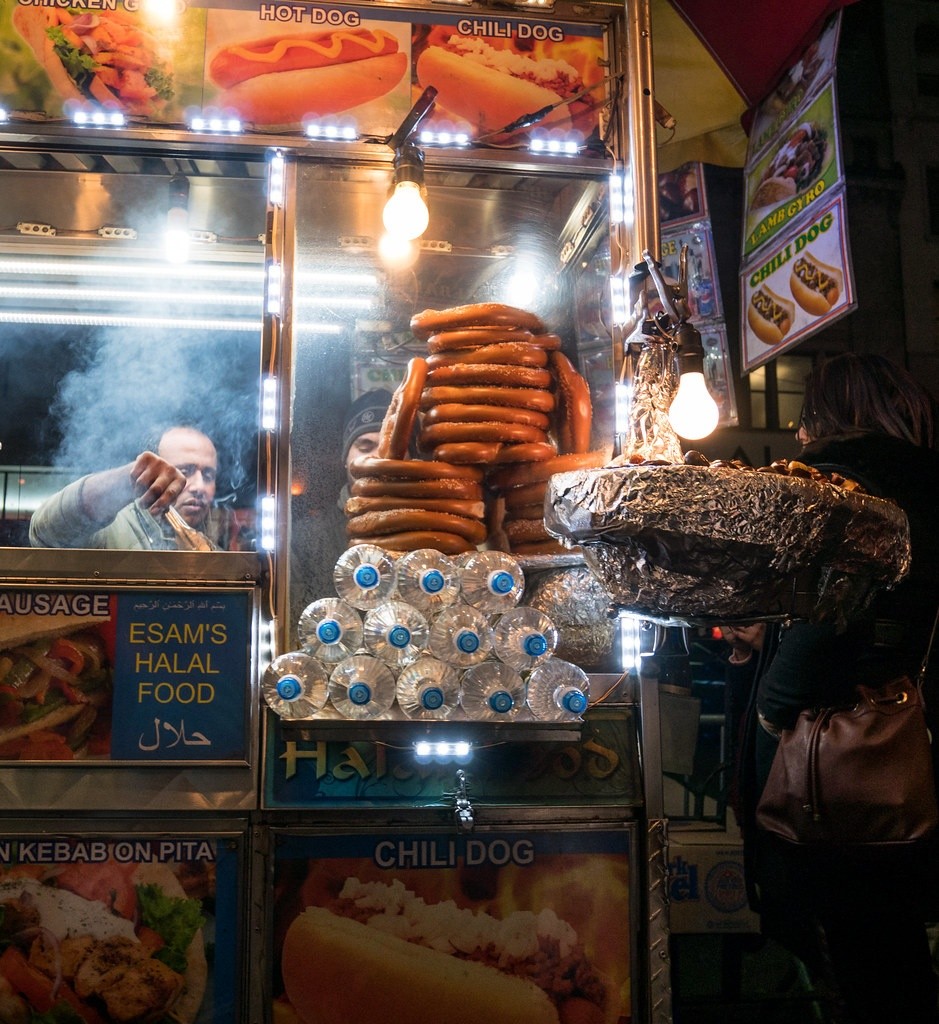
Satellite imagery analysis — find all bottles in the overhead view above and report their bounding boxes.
[424,604,494,669]
[263,649,330,719]
[329,648,396,720]
[484,608,558,670]
[449,551,525,614]
[394,549,461,614]
[333,543,398,611]
[362,602,428,667]
[455,653,525,723]
[628,341,684,464]
[297,598,363,664]
[519,656,591,723]
[396,653,461,721]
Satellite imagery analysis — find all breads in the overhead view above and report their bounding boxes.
[343,302,606,559]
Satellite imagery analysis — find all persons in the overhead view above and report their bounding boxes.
[289,387,421,654]
[30,423,224,552]
[713,334,939,1024]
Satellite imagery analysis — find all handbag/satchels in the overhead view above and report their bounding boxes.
[756,675,939,847]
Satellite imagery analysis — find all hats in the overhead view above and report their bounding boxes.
[341,388,419,463]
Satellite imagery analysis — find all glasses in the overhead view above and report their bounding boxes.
[798,411,817,428]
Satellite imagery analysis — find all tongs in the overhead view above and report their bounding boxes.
[163,504,216,551]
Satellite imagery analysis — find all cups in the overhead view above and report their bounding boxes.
[704,356,738,429]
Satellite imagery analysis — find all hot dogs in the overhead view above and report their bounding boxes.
[416,34,596,142]
[208,26,407,124]
[278,877,626,1024]
[747,284,795,345]
[790,252,842,315]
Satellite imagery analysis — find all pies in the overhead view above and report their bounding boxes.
[0,846,206,1024]
[750,125,833,209]
[10,1,176,116]
[0,595,112,744]
